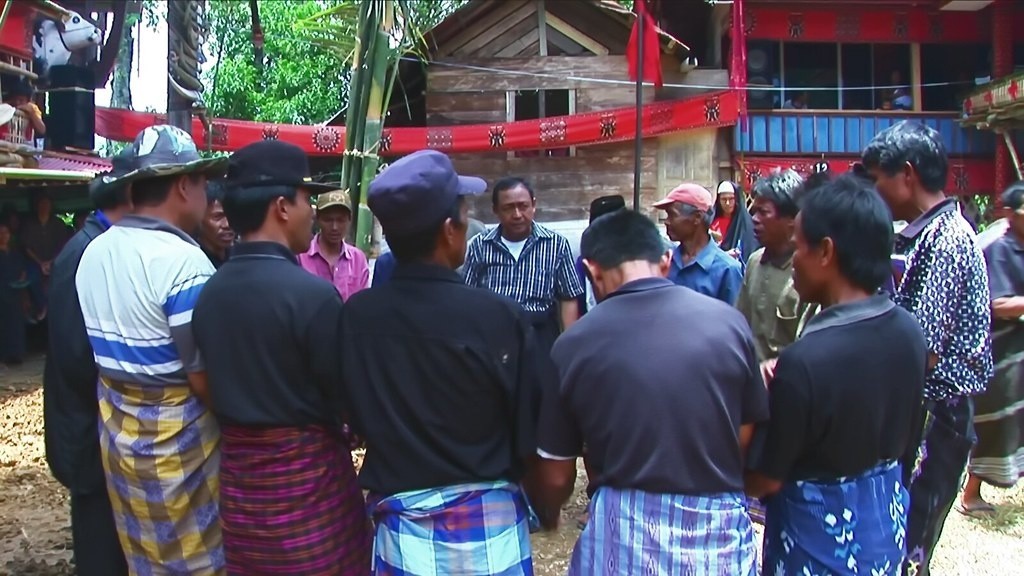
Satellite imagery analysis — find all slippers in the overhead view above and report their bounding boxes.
[954,496,1000,519]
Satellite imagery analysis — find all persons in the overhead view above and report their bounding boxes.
[651,184,744,308]
[0,192,92,345]
[294,186,370,303]
[339,147,546,576]
[193,178,240,270]
[575,193,626,317]
[879,68,914,110]
[457,176,584,529]
[733,172,822,391]
[784,88,810,110]
[73,123,224,576]
[533,208,769,575]
[465,215,487,241]
[191,140,375,576]
[708,176,762,272]
[862,119,1024,576]
[0,81,46,146]
[743,175,928,576]
[46,146,134,576]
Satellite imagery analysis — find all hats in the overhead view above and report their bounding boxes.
[368,149,487,232]
[718,182,735,193]
[225,140,342,194]
[653,183,713,212]
[317,189,351,214]
[102,124,225,183]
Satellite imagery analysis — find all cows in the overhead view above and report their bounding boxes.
[32,11,102,88]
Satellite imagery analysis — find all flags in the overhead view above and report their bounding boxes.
[629,1,662,91]
